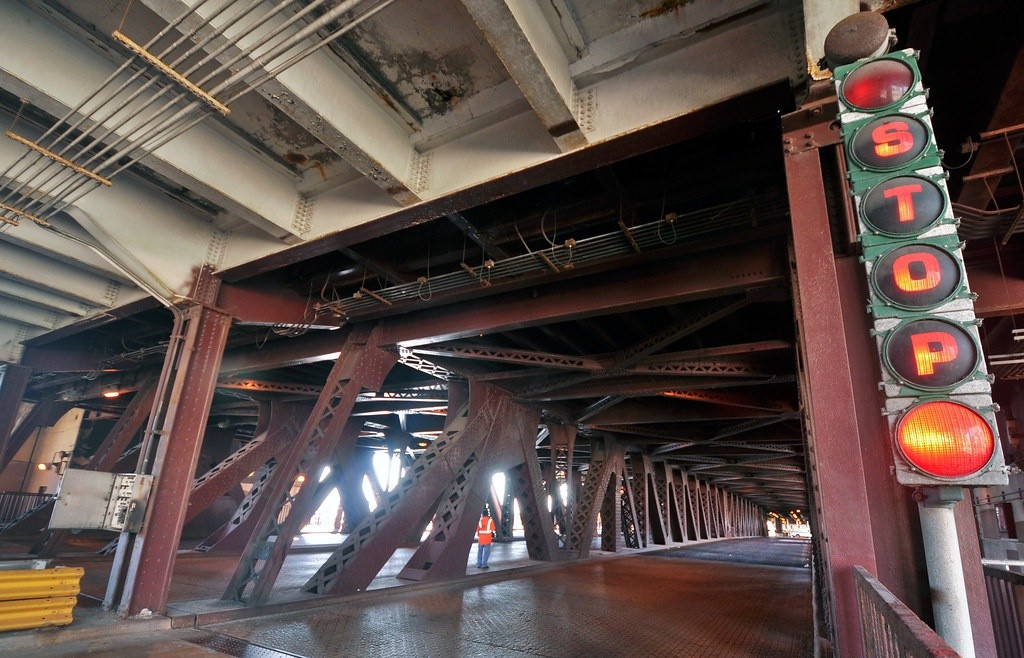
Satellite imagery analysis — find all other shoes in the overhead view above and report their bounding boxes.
[477,566,489,568]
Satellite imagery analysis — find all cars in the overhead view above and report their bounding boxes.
[791,530,813,538]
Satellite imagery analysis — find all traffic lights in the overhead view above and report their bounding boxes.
[832,47,1010,489]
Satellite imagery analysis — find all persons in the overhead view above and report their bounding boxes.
[477,508,496,569]
